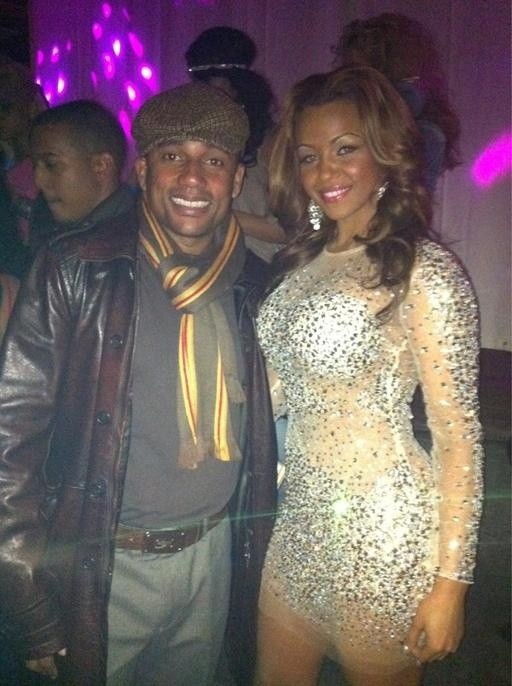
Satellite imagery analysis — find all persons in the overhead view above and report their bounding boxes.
[254,62,486,686]
[329,13,463,229]
[1,56,136,335]
[0,81,279,685]
[183,26,291,267]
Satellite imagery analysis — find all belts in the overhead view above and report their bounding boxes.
[111,504,229,555]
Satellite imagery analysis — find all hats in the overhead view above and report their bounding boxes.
[185,24,256,79]
[131,81,251,159]
